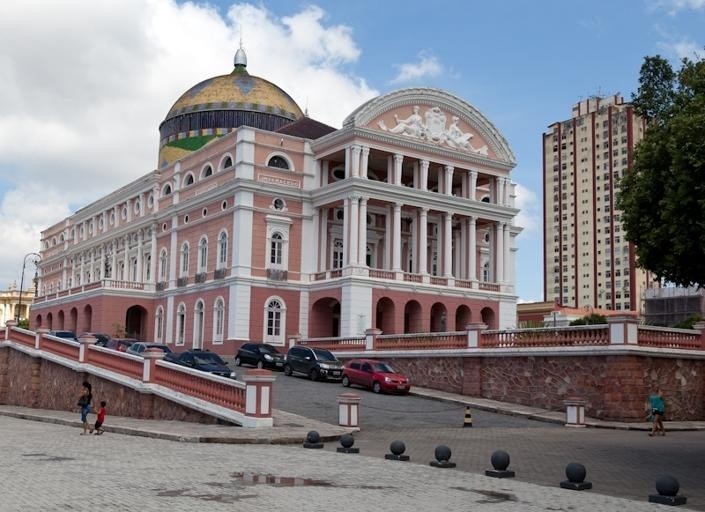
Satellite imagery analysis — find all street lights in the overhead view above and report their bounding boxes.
[17,252,41,324]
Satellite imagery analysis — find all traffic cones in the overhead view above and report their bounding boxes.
[462,406,473,428]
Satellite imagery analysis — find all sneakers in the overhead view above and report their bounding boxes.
[80,428,105,435]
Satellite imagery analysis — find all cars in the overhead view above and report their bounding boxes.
[49,330,236,381]
[235,342,411,395]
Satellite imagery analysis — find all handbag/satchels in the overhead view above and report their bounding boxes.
[76,396,89,406]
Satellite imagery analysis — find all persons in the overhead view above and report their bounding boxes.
[376,106,425,139]
[643,380,667,436]
[450,115,489,156]
[77,382,106,435]
